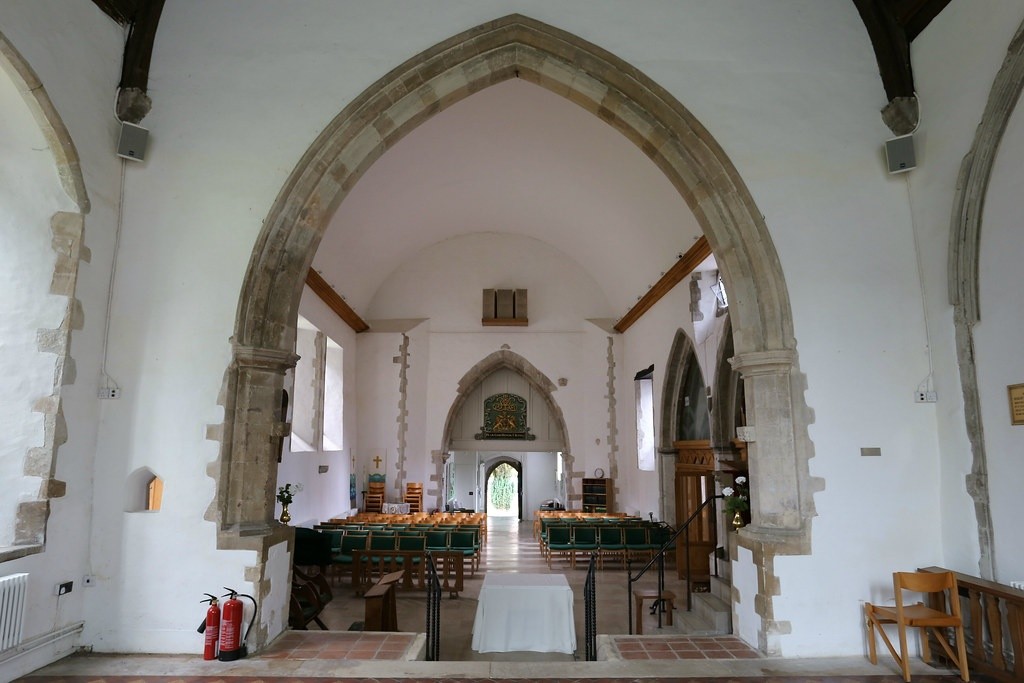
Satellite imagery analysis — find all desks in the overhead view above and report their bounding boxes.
[470,573,577,653]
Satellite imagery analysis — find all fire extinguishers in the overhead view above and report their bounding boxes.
[197,593,220,660]
[219,586,258,661]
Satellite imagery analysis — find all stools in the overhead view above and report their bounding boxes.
[633,589,670,633]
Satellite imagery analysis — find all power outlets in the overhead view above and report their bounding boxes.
[98,389,109,399]
[926,392,936,402]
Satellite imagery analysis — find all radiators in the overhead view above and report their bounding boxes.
[0,573,30,652]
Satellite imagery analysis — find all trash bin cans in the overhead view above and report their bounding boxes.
[540,499,565,511]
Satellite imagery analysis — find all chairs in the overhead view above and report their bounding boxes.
[533,510,676,569]
[308,512,488,576]
[403,482,423,512]
[364,474,387,512]
[864,570,970,683]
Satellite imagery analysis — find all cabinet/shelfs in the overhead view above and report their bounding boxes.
[581,478,614,513]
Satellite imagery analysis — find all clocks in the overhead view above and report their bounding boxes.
[594,468,604,478]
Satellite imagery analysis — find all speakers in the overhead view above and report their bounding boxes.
[117,121,149,162]
[884,133,917,175]
